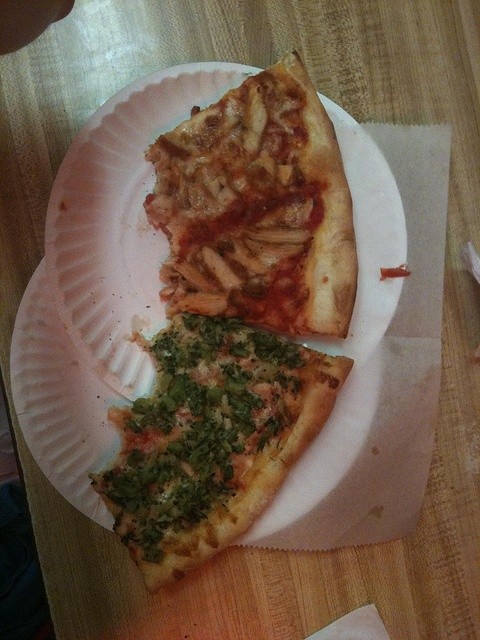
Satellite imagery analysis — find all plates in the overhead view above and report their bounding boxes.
[9,258,381,547]
[45,62,408,405]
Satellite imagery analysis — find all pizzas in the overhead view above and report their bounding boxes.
[144,53,360,339]
[91,314,355,595]
